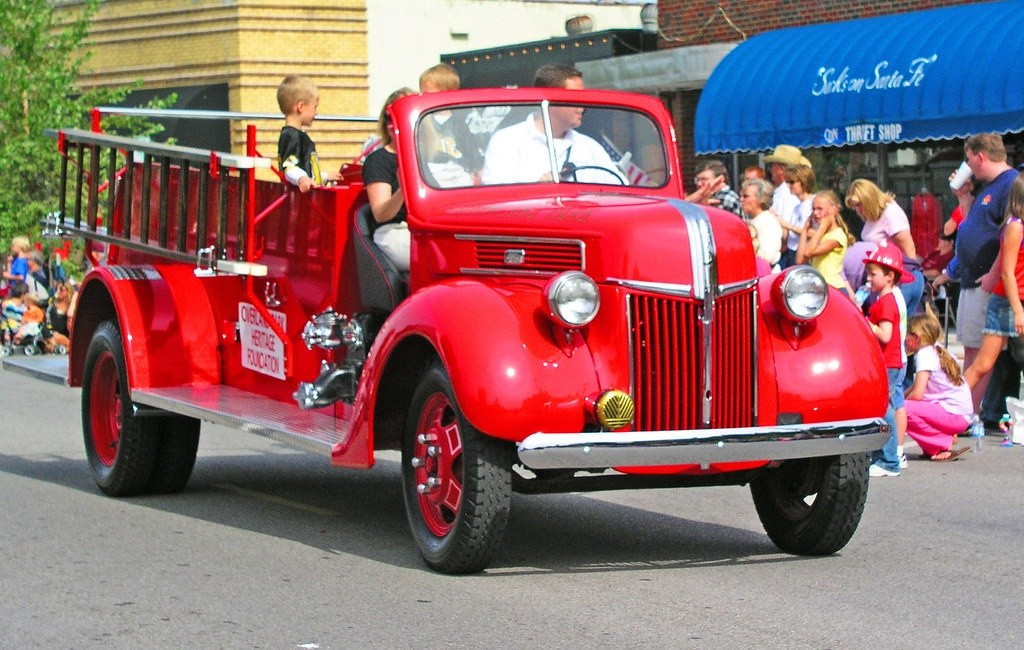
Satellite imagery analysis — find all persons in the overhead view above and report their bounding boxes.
[481,64,726,206]
[963,171,1024,394]
[842,241,924,390]
[762,144,812,216]
[861,243,914,477]
[932,133,1021,437]
[0,235,75,356]
[277,74,345,192]
[694,159,750,221]
[84,242,106,268]
[845,178,917,260]
[740,165,784,274]
[419,64,483,176]
[902,314,974,462]
[795,190,855,297]
[362,87,458,271]
[779,164,817,269]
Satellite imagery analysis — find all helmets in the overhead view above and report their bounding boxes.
[862,242,915,283]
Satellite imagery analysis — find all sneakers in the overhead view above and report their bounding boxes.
[868,463,900,478]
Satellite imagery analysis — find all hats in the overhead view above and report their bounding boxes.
[764,144,812,170]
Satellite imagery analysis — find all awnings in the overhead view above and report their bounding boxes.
[693,0,1024,157]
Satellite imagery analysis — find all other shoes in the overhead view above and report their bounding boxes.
[896,452,907,469]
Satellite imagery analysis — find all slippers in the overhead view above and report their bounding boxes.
[934,444,971,462]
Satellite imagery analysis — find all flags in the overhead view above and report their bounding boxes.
[601,139,651,186]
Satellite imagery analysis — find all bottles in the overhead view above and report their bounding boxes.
[853,281,871,307]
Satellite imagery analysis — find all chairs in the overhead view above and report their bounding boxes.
[353,200,411,313]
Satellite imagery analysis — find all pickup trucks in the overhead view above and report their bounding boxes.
[42,87,892,577]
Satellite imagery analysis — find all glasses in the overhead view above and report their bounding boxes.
[787,178,801,184]
[694,177,712,182]
